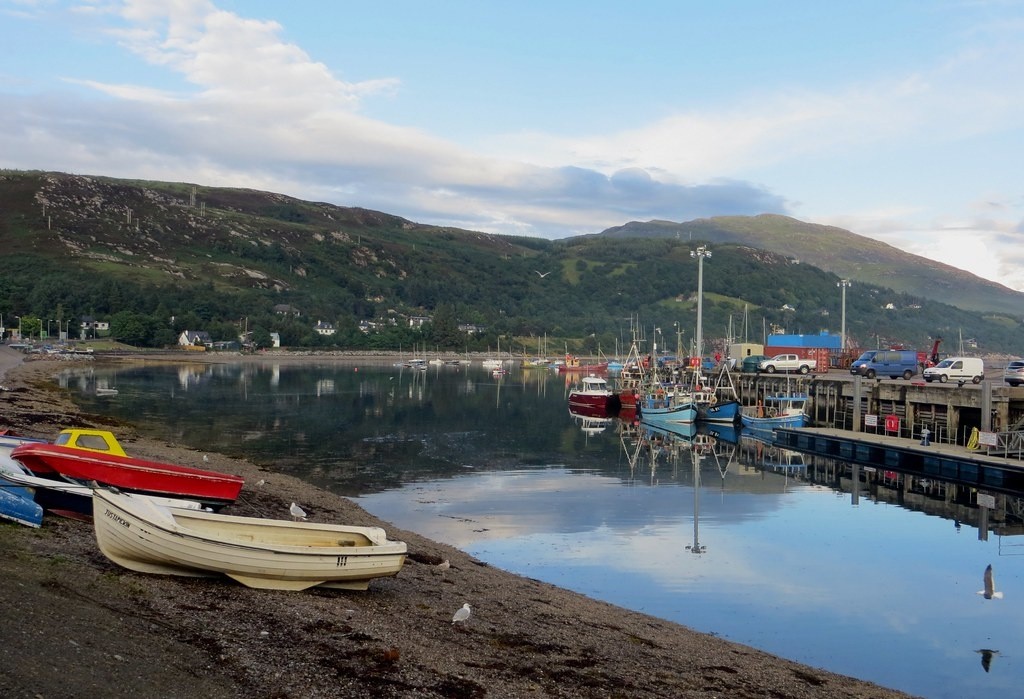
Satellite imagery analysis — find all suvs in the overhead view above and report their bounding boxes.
[1004,360,1024,387]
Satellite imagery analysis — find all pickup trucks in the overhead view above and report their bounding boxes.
[757,353,817,375]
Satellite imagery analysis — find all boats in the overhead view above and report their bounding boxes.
[8,426,246,514]
[0,469,215,523]
[91,479,408,592]
[0,486,44,529]
[0,435,48,467]
[7,343,94,355]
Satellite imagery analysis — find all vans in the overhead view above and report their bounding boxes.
[923,357,985,384]
[849,349,917,380]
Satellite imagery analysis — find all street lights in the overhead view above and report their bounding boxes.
[47,319,53,337]
[15,316,22,339]
[56,320,61,340]
[836,279,851,369]
[93,321,98,339]
[66,320,71,340]
[690,245,711,356]
[37,319,42,341]
[244,317,249,343]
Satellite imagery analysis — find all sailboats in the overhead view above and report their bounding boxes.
[394,303,806,450]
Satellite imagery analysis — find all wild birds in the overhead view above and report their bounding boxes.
[202,454,210,463]
[451,603,473,626]
[972,649,1003,673]
[433,559,451,577]
[975,563,1003,600]
[535,270,551,278]
[290,502,307,521]
[254,478,265,489]
